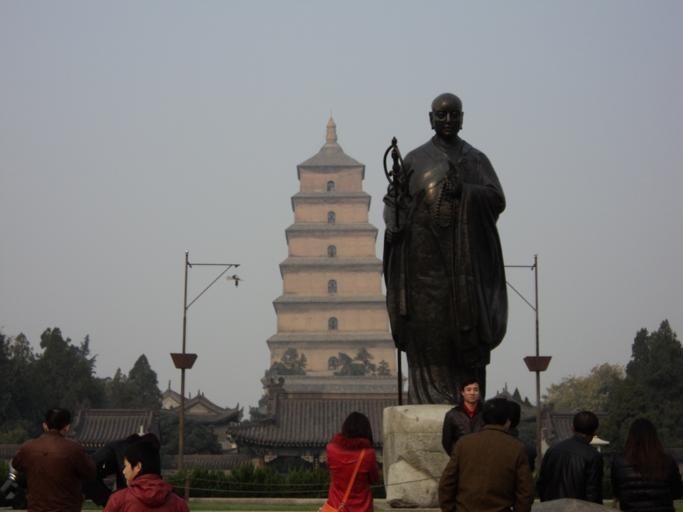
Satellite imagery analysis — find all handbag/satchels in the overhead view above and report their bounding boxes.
[318,500,342,512]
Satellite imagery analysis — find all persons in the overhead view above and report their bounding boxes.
[534,410,603,502]
[379,90,506,409]
[10,406,115,512]
[436,396,537,512]
[440,375,485,459]
[322,410,380,512]
[504,399,536,473]
[99,432,191,512]
[608,415,681,512]
